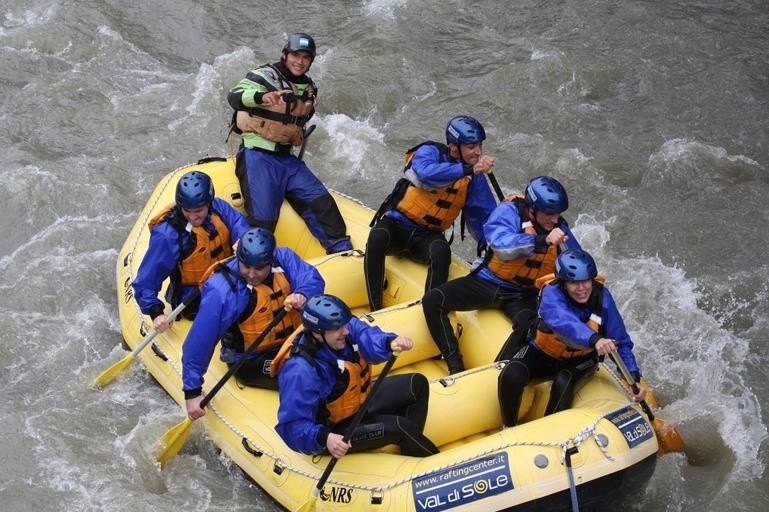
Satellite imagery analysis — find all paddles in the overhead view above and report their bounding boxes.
[296,348,402,512]
[156,303,290,473]
[91,284,200,398]
[560,240,660,410]
[610,346,683,458]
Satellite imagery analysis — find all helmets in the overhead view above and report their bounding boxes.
[553,248,597,281]
[175,170,214,208]
[283,32,316,57]
[523,176,568,215]
[445,114,486,145]
[302,293,351,330]
[235,227,276,267]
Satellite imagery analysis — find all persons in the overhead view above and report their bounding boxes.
[227,33,354,255]
[421,175,583,377]
[362,114,523,314]
[275,293,441,457]
[498,247,649,431]
[131,171,254,333]
[180,229,327,422]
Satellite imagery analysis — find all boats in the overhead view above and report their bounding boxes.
[116,158,658,512]
